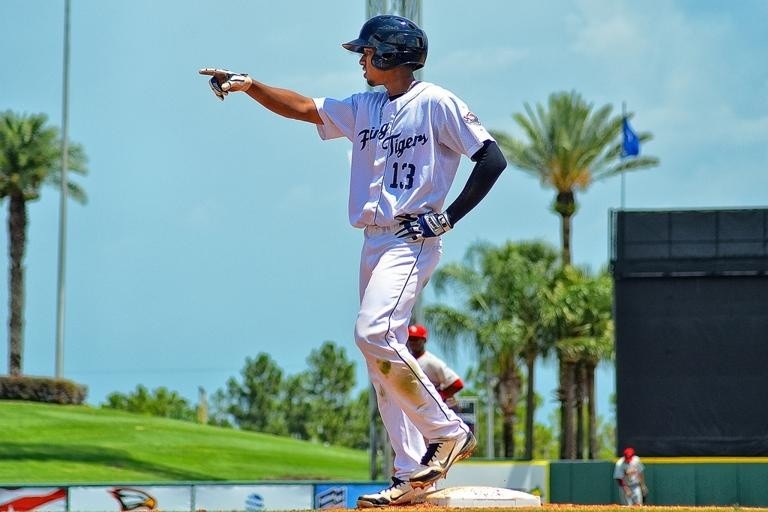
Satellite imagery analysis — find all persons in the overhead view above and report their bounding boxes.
[612,447,650,505]
[405,323,465,448]
[198,12,508,509]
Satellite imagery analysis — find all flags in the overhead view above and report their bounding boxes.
[621,117,639,158]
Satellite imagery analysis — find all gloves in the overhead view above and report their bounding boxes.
[391,209,454,243]
[197,66,253,102]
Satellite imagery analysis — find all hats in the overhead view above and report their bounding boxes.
[622,447,635,464]
[406,322,428,340]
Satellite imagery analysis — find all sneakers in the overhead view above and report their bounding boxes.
[355,475,437,509]
[407,429,478,491]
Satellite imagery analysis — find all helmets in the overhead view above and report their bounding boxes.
[340,14,428,72]
